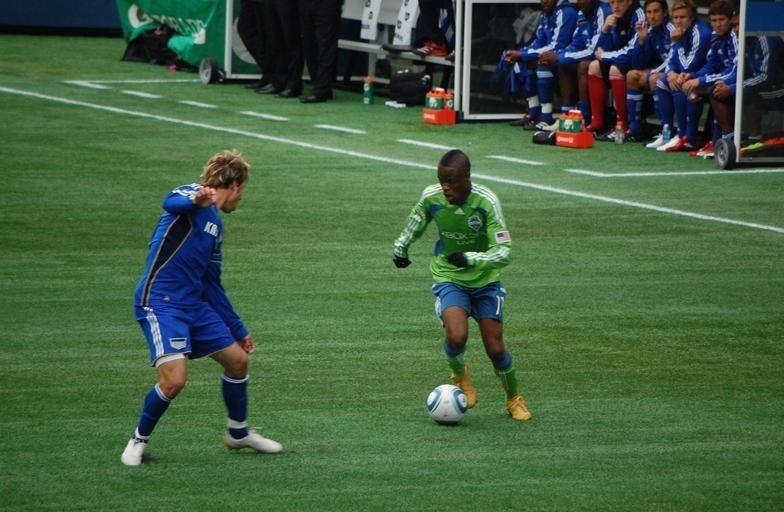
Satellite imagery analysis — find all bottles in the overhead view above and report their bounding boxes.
[662,124,671,145]
[423,91,454,109]
[362,76,374,106]
[614,121,625,145]
[557,108,585,134]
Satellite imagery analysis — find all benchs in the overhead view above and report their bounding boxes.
[332,1,478,106]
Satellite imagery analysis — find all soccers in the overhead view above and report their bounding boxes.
[426,384,468,422]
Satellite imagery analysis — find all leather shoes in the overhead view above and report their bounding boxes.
[245,80,333,102]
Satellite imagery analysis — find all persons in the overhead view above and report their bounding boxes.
[393,149,531,421]
[118,148,282,469]
[414,0,458,59]
[236,1,345,102]
[503,0,772,157]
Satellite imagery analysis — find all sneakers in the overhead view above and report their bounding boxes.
[646,130,724,157]
[505,395,532,420]
[412,42,447,56]
[450,365,477,409]
[224,426,283,453]
[120,427,151,466]
[509,115,641,143]
[741,139,764,156]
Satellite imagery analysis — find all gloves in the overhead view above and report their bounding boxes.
[447,252,469,268]
[393,256,412,268]
